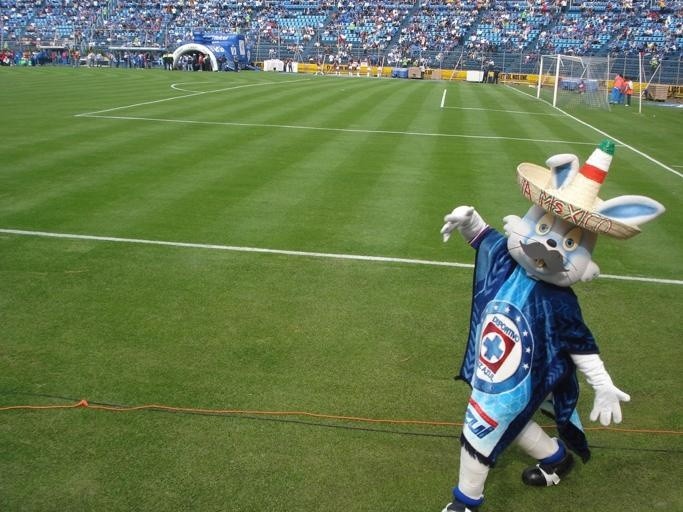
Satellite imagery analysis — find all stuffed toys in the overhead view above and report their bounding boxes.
[438,140,665,512]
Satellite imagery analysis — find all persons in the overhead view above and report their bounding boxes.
[0,1,476,80]
[475,0,680,108]
